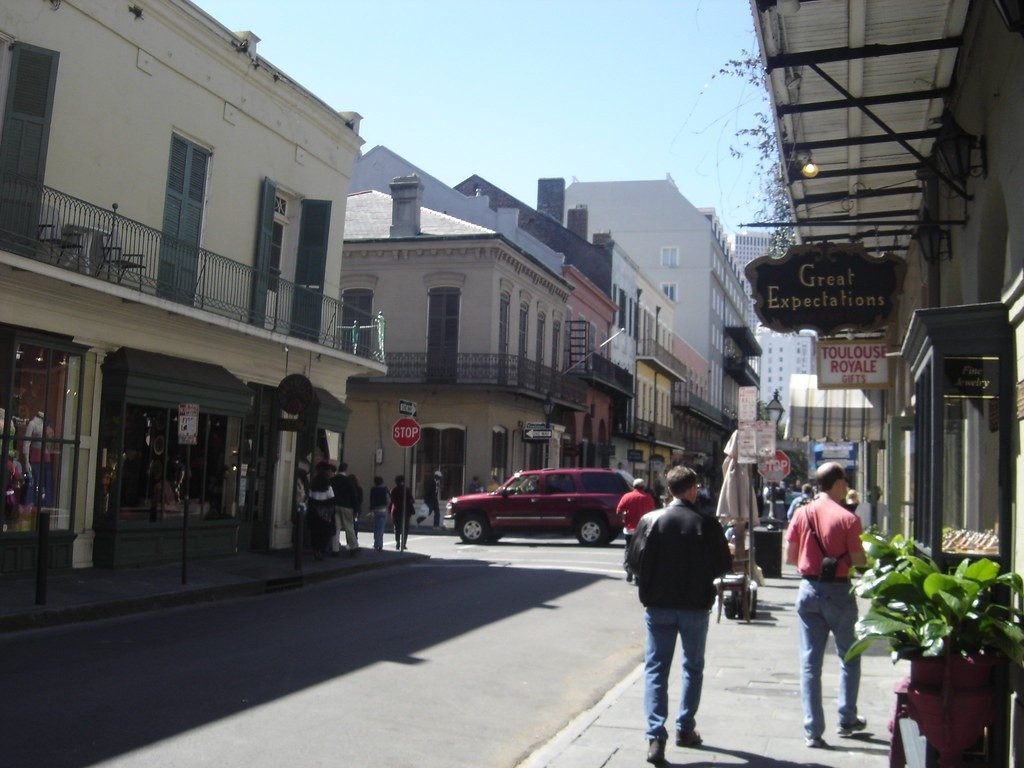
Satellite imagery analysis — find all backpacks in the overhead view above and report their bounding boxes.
[796,498,810,507]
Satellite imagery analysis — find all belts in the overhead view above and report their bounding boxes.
[801,575,847,583]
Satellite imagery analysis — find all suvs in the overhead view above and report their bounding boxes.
[443,466,636,546]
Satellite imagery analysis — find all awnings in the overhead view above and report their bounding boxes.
[748,0,970,262]
[683,451,707,465]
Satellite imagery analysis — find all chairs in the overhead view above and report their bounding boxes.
[92,224,143,292]
[35,204,82,272]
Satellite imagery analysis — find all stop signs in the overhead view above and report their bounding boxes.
[392,418,421,448]
[758,451,790,482]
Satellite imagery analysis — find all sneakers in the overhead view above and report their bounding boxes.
[806,735,822,747]
[837,716,867,736]
[647,740,665,762]
[676,728,700,745]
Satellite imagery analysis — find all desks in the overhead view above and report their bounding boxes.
[60,224,111,275]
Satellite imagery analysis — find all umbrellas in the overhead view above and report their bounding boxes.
[716,429,759,565]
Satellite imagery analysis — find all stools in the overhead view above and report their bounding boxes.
[891,679,1006,768]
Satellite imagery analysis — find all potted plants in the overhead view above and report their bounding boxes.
[844,528,1024,690]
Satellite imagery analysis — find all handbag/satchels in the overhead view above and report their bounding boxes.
[385,492,392,505]
[419,504,429,518]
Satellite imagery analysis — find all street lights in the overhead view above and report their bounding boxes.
[646,427,654,490]
[544,391,555,467]
[765,389,784,531]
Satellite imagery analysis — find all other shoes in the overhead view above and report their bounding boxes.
[626,569,634,583]
[397,542,407,549]
[315,551,324,561]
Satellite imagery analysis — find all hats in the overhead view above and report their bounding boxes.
[632,478,645,487]
[846,490,858,505]
[435,471,442,476]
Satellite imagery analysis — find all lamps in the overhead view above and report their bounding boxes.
[16,344,23,359]
[910,206,951,262]
[931,108,992,182]
[787,73,802,91]
[872,226,885,257]
[36,348,44,361]
[59,352,68,365]
[798,149,817,176]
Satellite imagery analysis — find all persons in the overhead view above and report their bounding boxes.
[388,474,415,549]
[369,476,391,551]
[784,462,867,747]
[623,466,733,761]
[616,478,654,585]
[151,455,185,506]
[416,471,443,531]
[307,460,364,561]
[0,408,55,532]
[468,475,500,494]
